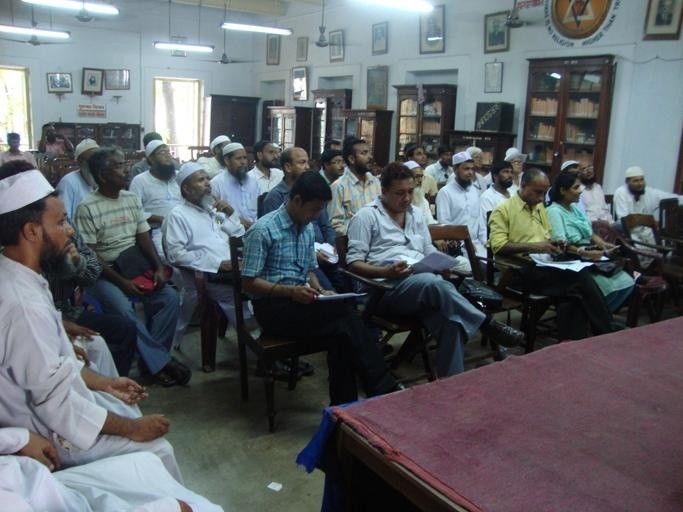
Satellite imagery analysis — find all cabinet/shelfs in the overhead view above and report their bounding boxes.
[267,105,313,152]
[42,122,143,149]
[522,54,619,186]
[341,110,394,168]
[311,88,352,162]
[392,84,457,164]
[203,95,261,152]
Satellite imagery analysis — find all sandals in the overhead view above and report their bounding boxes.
[257,358,312,379]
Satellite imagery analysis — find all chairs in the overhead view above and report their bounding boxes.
[24,145,682,432]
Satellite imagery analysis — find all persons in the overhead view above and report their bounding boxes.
[0,123,683,409]
[488,19,505,46]
[655,0,674,25]
[0,159,223,512]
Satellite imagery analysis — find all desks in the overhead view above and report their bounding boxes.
[447,129,517,166]
[297,316,683,511]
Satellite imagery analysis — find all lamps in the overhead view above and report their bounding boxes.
[152,0,214,53]
[22,0,120,15]
[219,1,293,36]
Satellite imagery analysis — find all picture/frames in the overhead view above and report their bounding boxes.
[266,34,308,102]
[418,4,444,54]
[171,35,187,57]
[644,1,683,40]
[46,66,131,94]
[483,10,509,93]
[365,21,388,110]
[328,29,343,62]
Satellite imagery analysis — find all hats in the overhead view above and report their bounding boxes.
[404,160,421,169]
[210,135,245,156]
[176,162,204,186]
[453,152,473,165]
[626,167,642,178]
[504,148,527,161]
[146,139,165,157]
[0,169,55,214]
[75,138,99,159]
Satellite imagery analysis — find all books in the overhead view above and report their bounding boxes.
[529,93,600,170]
[398,98,442,156]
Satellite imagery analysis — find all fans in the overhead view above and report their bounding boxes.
[293,0,367,49]
[198,0,256,64]
[50,0,137,25]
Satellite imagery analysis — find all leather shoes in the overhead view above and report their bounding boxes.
[155,355,191,386]
[486,320,525,346]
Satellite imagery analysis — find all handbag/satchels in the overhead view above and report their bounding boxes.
[114,244,172,293]
[448,277,503,310]
[580,246,626,277]
